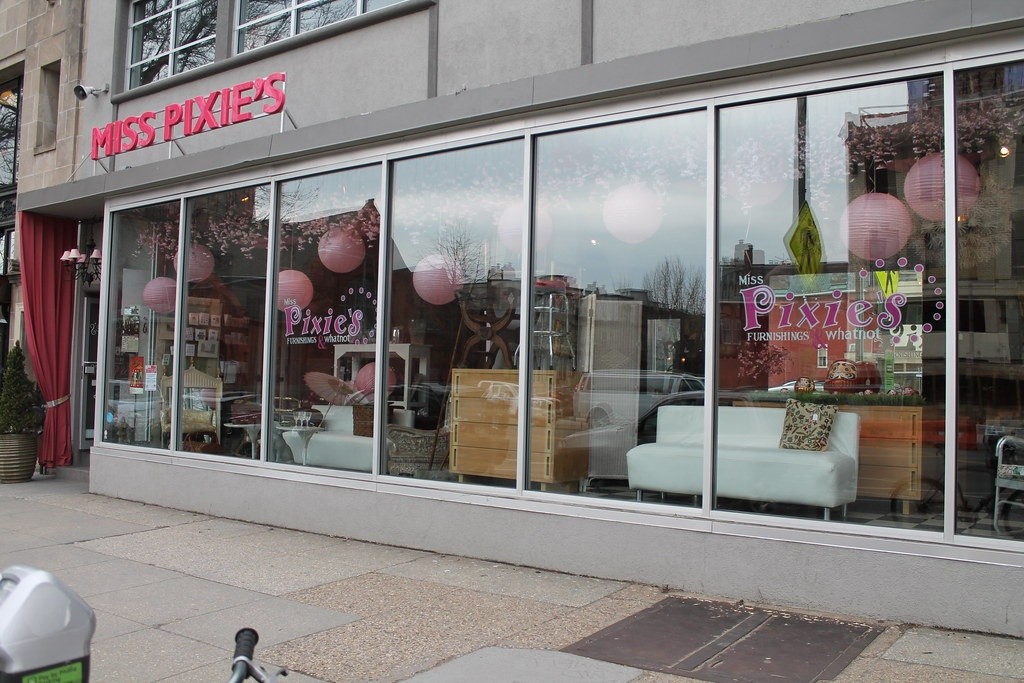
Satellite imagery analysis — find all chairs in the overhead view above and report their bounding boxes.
[159,365,222,451]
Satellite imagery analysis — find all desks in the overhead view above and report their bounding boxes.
[453,282,520,368]
[333,343,433,410]
[224,423,261,460]
[276,426,324,466]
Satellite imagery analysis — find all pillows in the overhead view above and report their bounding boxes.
[778,399,838,451]
[161,408,216,432]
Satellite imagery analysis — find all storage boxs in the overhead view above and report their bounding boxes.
[353,402,394,437]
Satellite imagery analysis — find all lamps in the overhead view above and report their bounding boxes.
[59,219,102,288]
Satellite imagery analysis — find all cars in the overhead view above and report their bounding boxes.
[107,378,324,444]
[345,385,446,431]
[767,380,825,393]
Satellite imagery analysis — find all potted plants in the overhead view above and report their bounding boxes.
[0,340,45,485]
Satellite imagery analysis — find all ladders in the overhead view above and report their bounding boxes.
[440,291,540,472]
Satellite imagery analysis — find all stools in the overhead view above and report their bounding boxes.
[513,281,577,371]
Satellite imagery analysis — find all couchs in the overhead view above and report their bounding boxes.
[282,405,416,473]
[626,398,860,522]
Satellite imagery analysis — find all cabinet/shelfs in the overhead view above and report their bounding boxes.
[732,391,939,515]
[993,434,1024,537]
[448,368,590,493]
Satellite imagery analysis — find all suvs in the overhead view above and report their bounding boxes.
[574,367,707,431]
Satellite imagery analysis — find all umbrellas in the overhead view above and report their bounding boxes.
[303,372,370,434]
